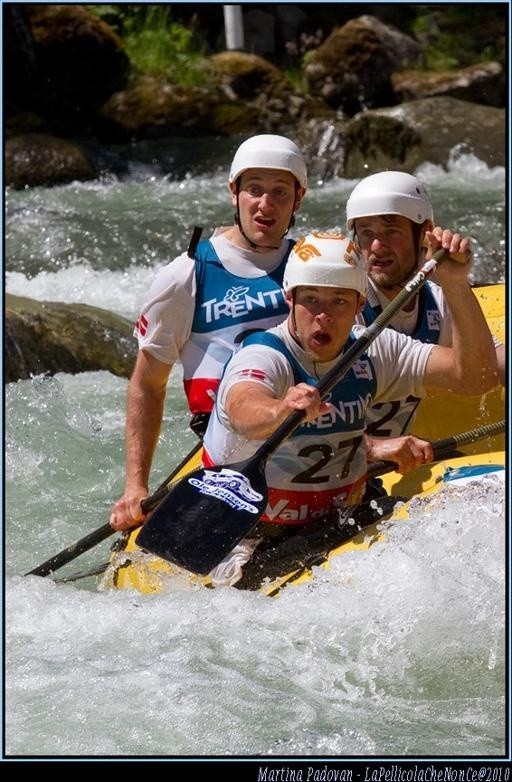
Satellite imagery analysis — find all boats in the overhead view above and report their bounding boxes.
[102,280,506,601]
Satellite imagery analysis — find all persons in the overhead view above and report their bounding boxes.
[109,134,307,533]
[343,169,508,500]
[200,218,498,592]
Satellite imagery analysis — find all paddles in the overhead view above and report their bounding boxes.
[136,231,472,576]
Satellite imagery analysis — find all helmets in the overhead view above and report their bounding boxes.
[227,133,308,189]
[282,230,368,299]
[345,171,435,232]
